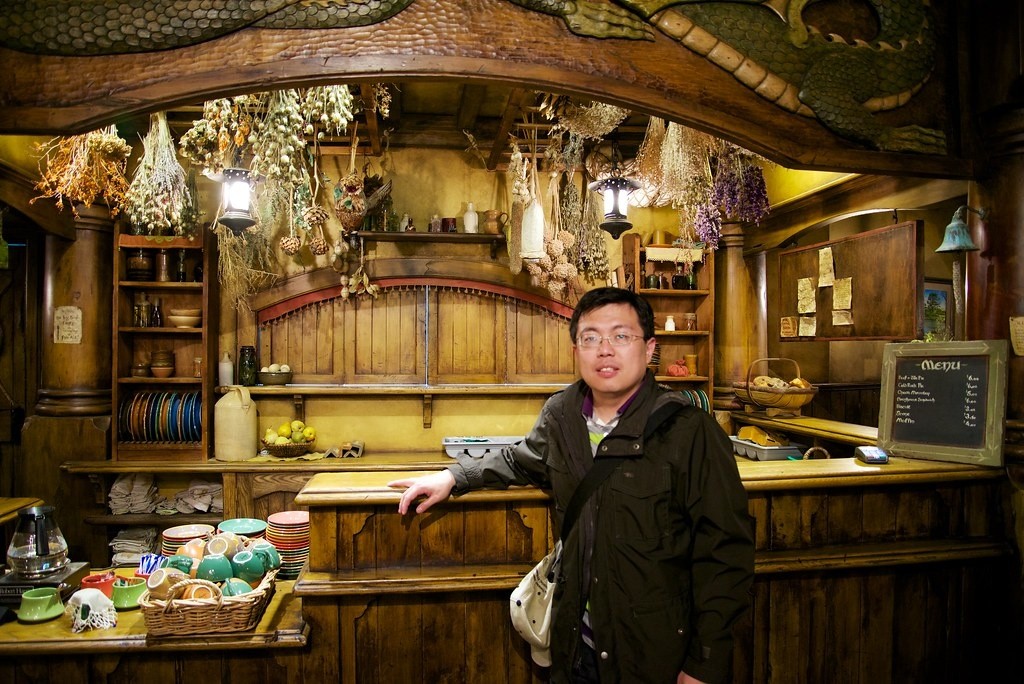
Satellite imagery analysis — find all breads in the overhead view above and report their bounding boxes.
[752,375,812,390]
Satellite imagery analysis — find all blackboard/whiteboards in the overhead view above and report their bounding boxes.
[876,339,1010,468]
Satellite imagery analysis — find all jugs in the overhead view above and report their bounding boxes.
[7,505,68,580]
[483,209,509,235]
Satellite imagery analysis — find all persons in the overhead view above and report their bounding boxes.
[387,287,757,684]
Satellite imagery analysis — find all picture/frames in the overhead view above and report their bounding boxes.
[924,276,955,341]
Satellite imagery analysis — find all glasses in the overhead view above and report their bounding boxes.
[573,332,647,348]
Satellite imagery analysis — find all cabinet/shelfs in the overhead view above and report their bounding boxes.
[622,233,713,417]
[59,460,237,567]
[111,208,218,461]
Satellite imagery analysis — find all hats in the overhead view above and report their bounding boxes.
[509,551,557,667]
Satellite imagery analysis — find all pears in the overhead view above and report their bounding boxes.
[265,419,314,445]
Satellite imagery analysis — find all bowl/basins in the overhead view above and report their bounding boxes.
[17,587,66,622]
[81,574,115,599]
[150,349,174,378]
[110,577,147,609]
[168,315,201,329]
[131,367,149,377]
[255,371,293,387]
[169,307,202,316]
[647,342,660,376]
[145,532,280,600]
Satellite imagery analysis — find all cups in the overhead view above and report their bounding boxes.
[441,217,457,232]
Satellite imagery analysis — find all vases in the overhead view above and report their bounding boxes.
[218,351,233,386]
[664,316,675,331]
[238,346,258,387]
[463,201,479,233]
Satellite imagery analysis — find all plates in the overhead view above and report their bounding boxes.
[440,434,528,458]
[161,511,310,580]
[119,391,203,441]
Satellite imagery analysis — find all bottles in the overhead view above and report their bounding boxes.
[193,357,202,377]
[150,297,163,328]
[237,345,257,387]
[176,250,187,282]
[646,266,696,290]
[405,218,416,232]
[399,212,409,232]
[219,351,233,386]
[156,250,171,282]
[428,214,441,233]
[683,313,696,331]
[133,293,153,327]
[128,250,151,281]
[213,384,258,462]
[463,203,479,233]
[664,316,675,332]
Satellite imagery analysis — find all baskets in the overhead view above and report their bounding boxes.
[734,358,819,410]
[141,551,284,637]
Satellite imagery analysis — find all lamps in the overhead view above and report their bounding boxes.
[587,124,642,240]
[934,205,990,252]
[217,144,256,236]
